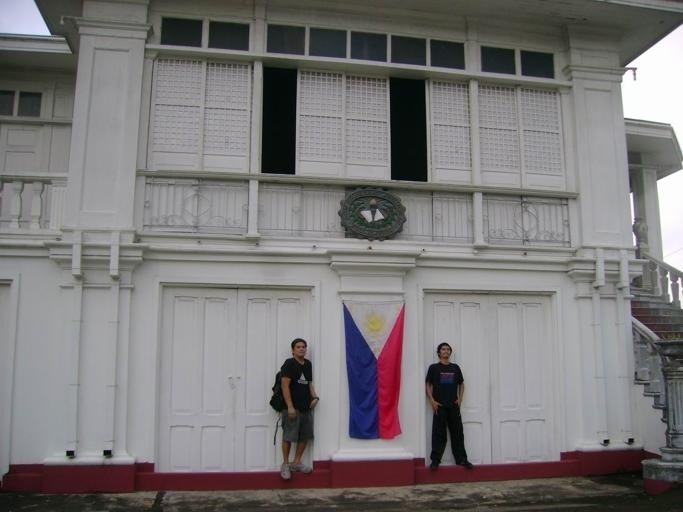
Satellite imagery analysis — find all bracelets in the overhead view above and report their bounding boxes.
[312,397,319,400]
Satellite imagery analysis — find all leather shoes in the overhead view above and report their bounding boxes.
[429,460,442,470]
[460,461,474,470]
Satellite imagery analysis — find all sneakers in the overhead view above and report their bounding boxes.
[291,463,313,475]
[279,462,291,481]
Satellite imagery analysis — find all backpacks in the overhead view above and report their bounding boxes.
[269,372,286,413]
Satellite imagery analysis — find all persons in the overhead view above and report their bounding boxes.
[280,338,319,480]
[425,343,473,470]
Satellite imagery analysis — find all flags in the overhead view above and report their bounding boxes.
[343,301,406,439]
